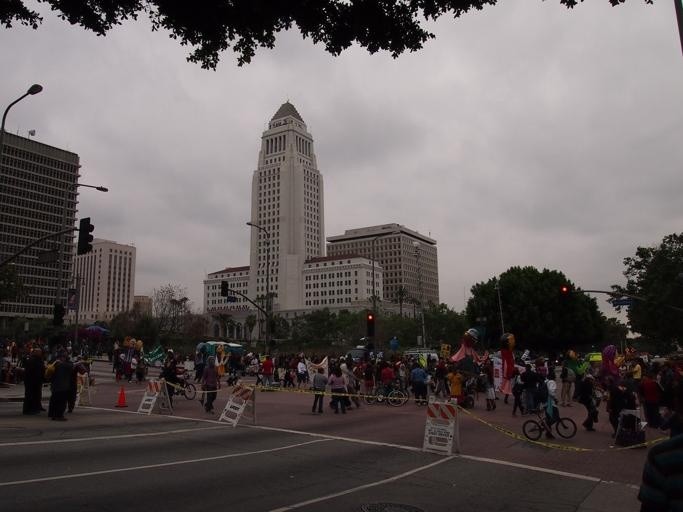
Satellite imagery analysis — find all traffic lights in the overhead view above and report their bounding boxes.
[77,217,94,256]
[561,285,570,295]
[54,305,65,326]
[365,312,374,338]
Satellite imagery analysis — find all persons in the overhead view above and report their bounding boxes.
[157,341,551,414]
[109,335,149,384]
[560,323,681,436]
[635,434,682,511]
[536,368,560,437]
[8,339,102,421]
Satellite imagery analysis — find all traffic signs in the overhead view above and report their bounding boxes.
[612,298,632,307]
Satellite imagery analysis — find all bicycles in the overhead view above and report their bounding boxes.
[173,369,196,399]
[522,404,577,441]
[364,383,410,406]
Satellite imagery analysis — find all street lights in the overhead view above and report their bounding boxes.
[0,84,43,148]
[244,222,270,354]
[493,286,504,334]
[372,230,401,311]
[54,183,108,302]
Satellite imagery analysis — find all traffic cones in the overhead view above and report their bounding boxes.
[114,386,129,408]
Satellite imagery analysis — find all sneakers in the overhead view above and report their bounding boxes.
[255,382,307,390]
[484,402,548,416]
[18,401,226,421]
[308,399,429,418]
[545,402,601,441]
